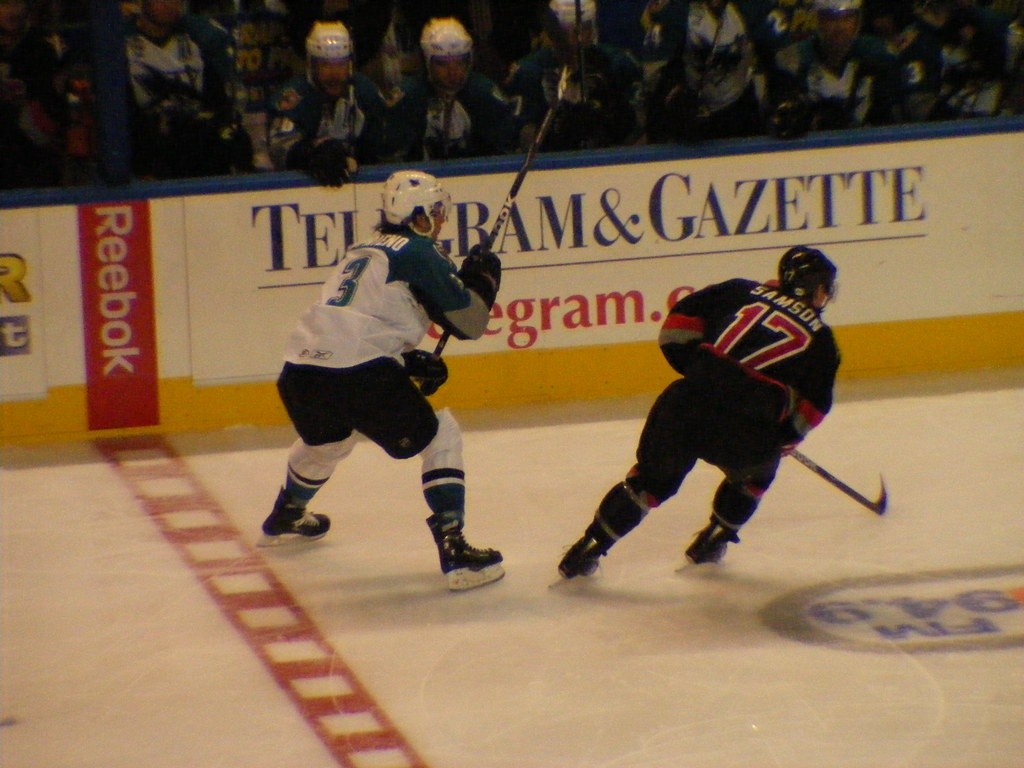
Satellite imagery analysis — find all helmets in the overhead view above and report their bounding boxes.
[420,17,474,73]
[549,0,598,40]
[305,20,355,78]
[778,245,838,298]
[815,0,863,46]
[383,169,447,225]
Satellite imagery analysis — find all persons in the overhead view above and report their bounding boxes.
[262,171,503,575]
[0,0,1024,191]
[558,246,841,580]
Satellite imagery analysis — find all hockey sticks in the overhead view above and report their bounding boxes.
[413,61,573,385]
[787,447,889,517]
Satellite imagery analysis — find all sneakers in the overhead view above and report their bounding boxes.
[256,486,330,546]
[547,534,610,588]
[672,514,741,572]
[427,514,505,591]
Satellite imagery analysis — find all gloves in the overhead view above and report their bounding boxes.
[400,347,448,396]
[779,418,804,458]
[303,136,358,188]
[455,245,502,311]
